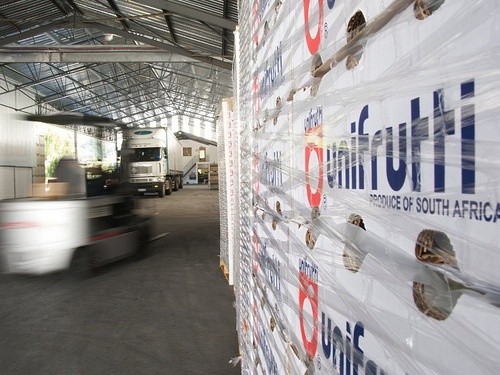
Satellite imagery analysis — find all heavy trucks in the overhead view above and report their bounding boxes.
[115,127,183,199]
